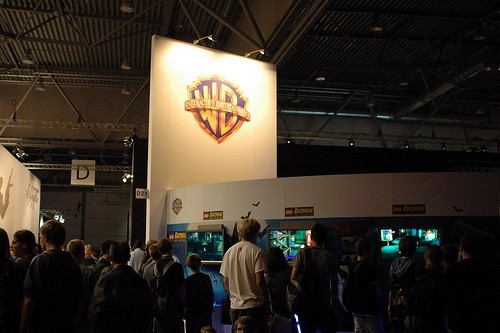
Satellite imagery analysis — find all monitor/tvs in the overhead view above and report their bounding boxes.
[268,228,314,264]
[185,229,226,264]
[379,228,441,259]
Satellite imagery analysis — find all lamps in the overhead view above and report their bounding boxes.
[121,56,132,70]
[36,79,45,91]
[120,0,134,13]
[22,50,33,64]
[193,35,213,44]
[245,49,264,57]
[316,73,325,80]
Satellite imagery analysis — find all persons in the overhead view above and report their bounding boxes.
[0,219,500,333]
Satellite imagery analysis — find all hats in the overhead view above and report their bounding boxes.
[399,237,417,257]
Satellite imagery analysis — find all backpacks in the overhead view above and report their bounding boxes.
[285,246,312,314]
[150,261,176,315]
